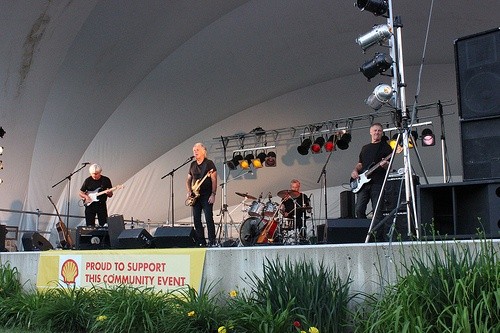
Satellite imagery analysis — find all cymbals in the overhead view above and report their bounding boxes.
[277,189,300,199]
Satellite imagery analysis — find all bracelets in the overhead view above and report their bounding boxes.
[212,192,216,195]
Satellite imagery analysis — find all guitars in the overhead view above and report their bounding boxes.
[185,169,213,206]
[256,192,290,244]
[46,195,73,249]
[349,146,402,195]
[83,184,128,206]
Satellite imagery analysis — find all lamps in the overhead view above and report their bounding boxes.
[353,0,389,18]
[297,138,312,155]
[264,151,276,167]
[311,137,324,153]
[358,53,393,81]
[389,133,403,150]
[356,22,391,53]
[336,133,352,151]
[251,152,267,168]
[227,155,244,170]
[322,135,338,152]
[241,154,254,170]
[407,130,419,148]
[364,82,393,110]
[421,128,436,146]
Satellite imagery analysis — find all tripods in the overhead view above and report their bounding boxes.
[272,192,310,245]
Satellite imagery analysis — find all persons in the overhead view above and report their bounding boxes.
[280,179,311,242]
[351,123,393,243]
[79,164,113,226]
[186,143,217,247]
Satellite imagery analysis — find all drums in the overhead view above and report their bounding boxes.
[280,218,294,229]
[235,192,257,200]
[262,201,281,217]
[239,216,271,246]
[248,200,265,217]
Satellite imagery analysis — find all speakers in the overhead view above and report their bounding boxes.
[453,26,500,182]
[316,175,419,244]
[154,226,203,248]
[21,232,54,251]
[75,226,110,250]
[118,228,154,249]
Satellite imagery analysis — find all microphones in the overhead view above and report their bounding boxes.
[81,161,91,164]
[334,129,349,134]
[188,155,195,159]
